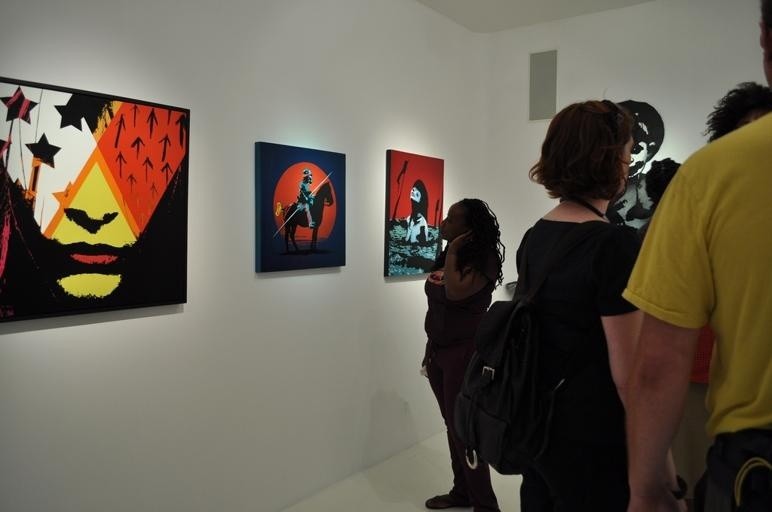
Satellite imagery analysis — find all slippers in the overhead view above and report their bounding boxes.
[425,487,501,512]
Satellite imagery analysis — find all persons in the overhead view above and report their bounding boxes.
[1,84,187,304]
[404,178,430,245]
[296,167,318,229]
[621,3,772,512]
[421,197,508,512]
[487,93,694,512]
[598,99,684,234]
[701,81,772,146]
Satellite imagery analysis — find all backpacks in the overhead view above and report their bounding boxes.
[451,221,607,477]
